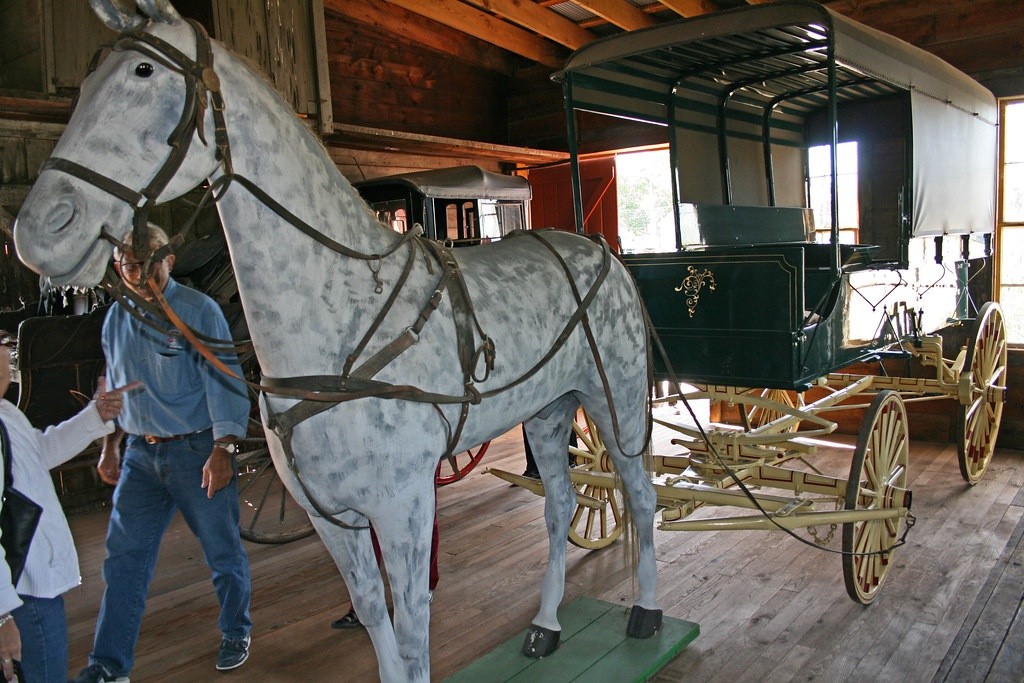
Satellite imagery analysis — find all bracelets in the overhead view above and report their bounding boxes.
[0,614,14,626]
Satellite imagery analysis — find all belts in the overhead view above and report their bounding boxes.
[140,428,211,445]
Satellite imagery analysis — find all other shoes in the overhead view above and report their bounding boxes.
[332,608,364,628]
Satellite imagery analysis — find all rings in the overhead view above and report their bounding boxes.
[1,658,11,665]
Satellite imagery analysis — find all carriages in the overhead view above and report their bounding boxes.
[13,0,1010,683]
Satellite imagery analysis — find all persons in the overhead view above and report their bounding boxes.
[71,220,254,683]
[0,331,142,683]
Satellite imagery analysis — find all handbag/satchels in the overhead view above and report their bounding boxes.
[0,417,44,589]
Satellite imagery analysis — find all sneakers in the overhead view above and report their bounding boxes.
[215,637,251,669]
[74,665,131,683]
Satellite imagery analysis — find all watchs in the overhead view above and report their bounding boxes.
[213,441,238,454]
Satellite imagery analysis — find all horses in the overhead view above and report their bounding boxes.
[14,1,662,682]
